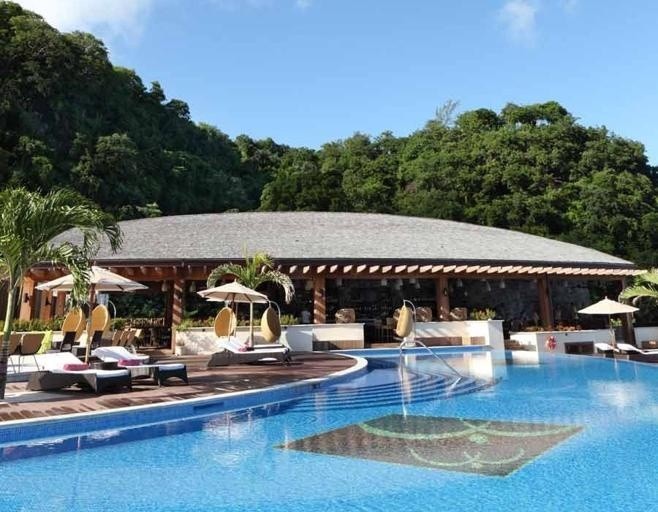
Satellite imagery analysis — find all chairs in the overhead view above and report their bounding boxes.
[380,317,395,343]
[592,340,657,365]
[0,319,288,391]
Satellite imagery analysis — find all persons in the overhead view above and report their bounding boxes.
[554,301,581,323]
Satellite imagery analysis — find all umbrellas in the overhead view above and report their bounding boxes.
[35,265,149,363]
[196,278,269,340]
[577,294,640,353]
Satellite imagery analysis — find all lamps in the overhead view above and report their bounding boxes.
[302,275,343,291]
[161,275,200,292]
[454,276,507,292]
[380,276,421,292]
